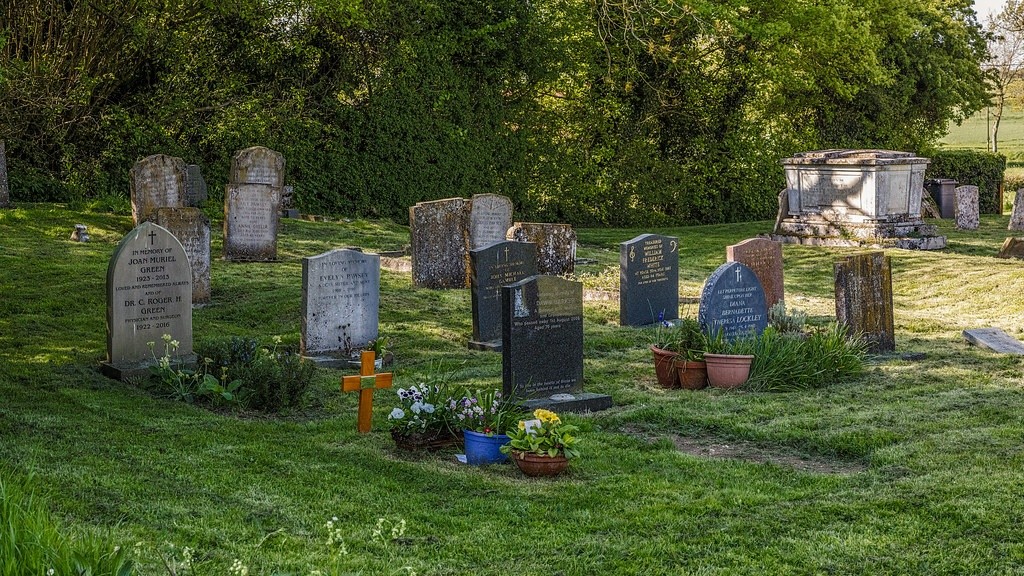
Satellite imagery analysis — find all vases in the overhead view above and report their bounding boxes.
[388,421,452,453]
[462,429,512,466]
[649,347,679,390]
[511,451,568,478]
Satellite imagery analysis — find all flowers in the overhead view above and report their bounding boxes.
[651,301,676,348]
[384,356,458,430]
[449,382,533,432]
[497,409,580,461]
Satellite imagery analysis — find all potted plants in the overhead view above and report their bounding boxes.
[704,329,754,387]
[674,317,710,389]
